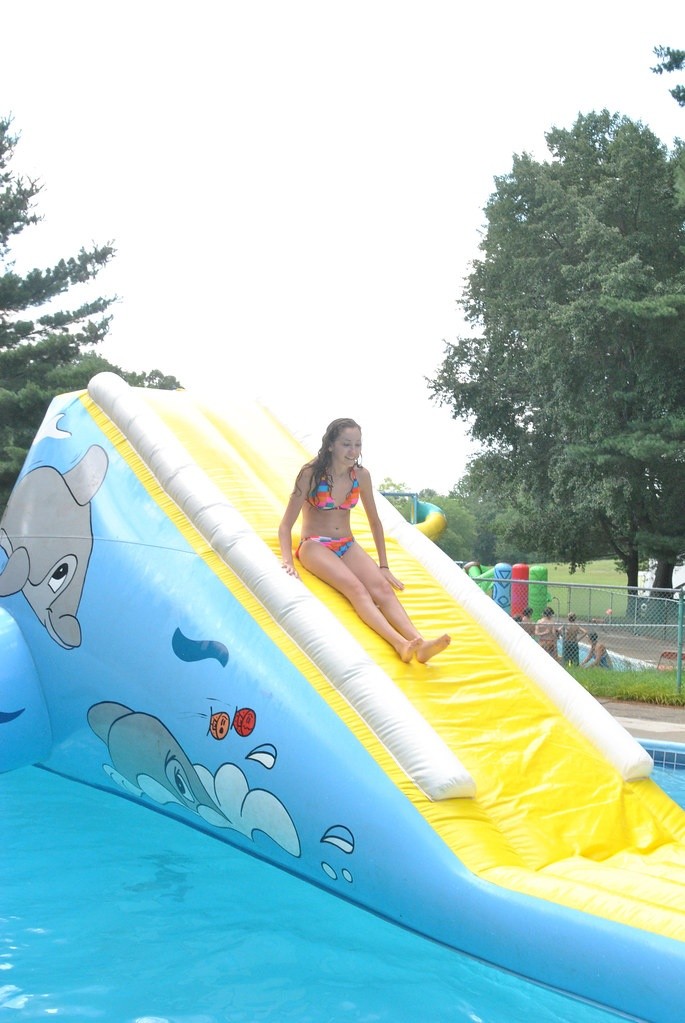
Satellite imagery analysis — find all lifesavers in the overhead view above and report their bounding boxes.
[640,603,648,611]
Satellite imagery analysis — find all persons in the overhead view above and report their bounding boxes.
[513,607,613,670]
[278,418,451,664]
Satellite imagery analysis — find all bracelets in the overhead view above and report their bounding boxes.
[379,566,390,569]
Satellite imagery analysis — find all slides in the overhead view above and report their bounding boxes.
[51,371,685,881]
